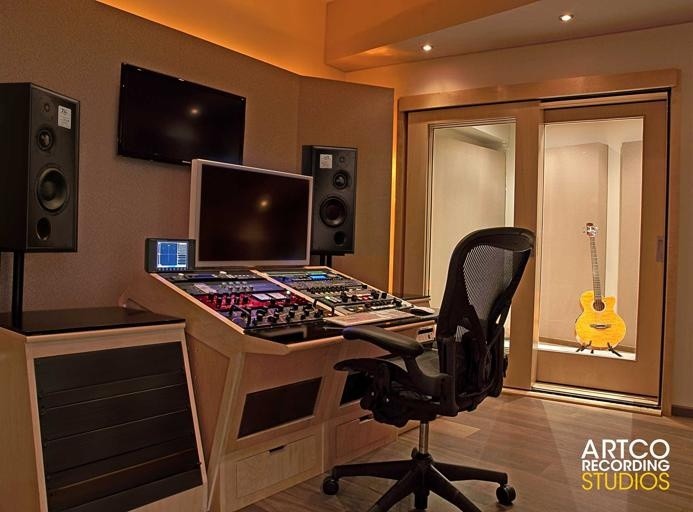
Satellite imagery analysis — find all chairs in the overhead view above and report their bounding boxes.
[318,224,538,512]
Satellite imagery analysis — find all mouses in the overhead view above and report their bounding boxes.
[410,305,435,314]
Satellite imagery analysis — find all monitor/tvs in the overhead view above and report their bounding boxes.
[188,156,315,269]
[116,60,248,167]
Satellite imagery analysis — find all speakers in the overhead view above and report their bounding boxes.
[301,143,359,256]
[0,81,80,254]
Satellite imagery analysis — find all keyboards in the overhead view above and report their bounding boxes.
[322,308,417,329]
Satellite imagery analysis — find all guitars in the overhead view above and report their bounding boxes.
[575,221,627,350]
